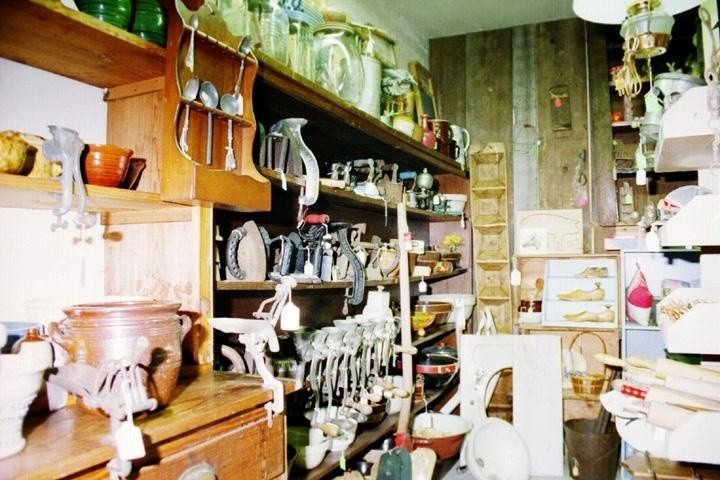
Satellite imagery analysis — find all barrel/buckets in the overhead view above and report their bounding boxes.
[563,418,621,478]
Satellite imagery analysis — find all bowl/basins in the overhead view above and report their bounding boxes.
[413,294,476,330]
[77,143,133,187]
[444,194,468,212]
[287,355,473,475]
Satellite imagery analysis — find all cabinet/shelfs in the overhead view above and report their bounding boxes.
[1,1,703,480]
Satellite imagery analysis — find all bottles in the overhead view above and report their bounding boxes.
[420,113,435,150]
[288,20,364,105]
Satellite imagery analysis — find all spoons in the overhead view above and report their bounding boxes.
[235,34,252,100]
[188,13,201,71]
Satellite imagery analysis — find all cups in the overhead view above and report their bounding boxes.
[431,120,460,159]
